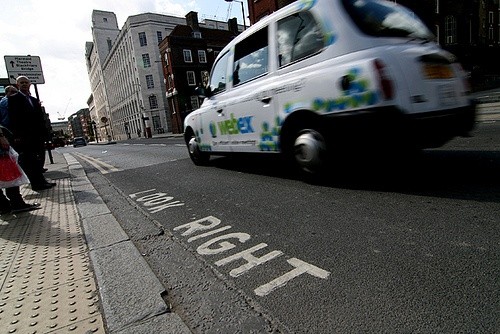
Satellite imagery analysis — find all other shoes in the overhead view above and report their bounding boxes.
[0,194,41,214]
[42,168,48,172]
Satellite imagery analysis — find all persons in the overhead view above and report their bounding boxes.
[26,89,48,174]
[0,85,20,123]
[0,125,43,215]
[7,75,57,192]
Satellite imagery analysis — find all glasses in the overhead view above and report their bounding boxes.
[8,91,17,94]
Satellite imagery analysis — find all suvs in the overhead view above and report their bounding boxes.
[73,137,86,147]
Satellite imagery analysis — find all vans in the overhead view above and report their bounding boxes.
[183,0,471,174]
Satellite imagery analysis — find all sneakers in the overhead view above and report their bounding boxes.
[32,180,56,190]
